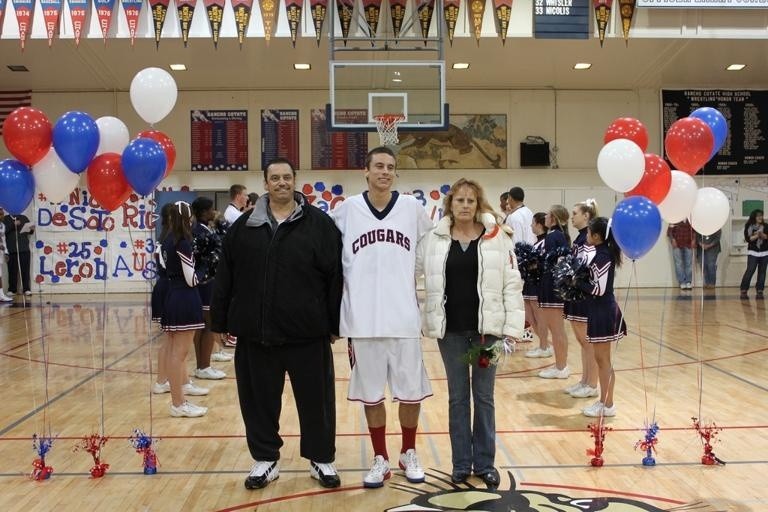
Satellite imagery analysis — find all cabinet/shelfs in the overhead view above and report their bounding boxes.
[730,217,768,256]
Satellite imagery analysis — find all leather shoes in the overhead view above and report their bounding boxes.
[452,471,497,485]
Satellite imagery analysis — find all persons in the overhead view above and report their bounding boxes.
[151,183,262,417]
[667,220,696,289]
[1,214,35,296]
[695,228,722,290]
[739,209,768,299]
[1,209,14,303]
[414,177,525,485]
[208,158,343,490]
[323,147,438,489]
[501,187,628,418]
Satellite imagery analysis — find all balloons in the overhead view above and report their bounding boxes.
[596,105,730,262]
[1,66,179,216]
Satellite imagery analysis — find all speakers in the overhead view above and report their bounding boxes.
[520,142,550,167]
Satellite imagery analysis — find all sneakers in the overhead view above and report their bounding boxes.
[153,381,209,417]
[539,366,568,379]
[310,461,340,488]
[245,461,279,490]
[364,449,425,488]
[196,351,233,379]
[583,401,616,417]
[526,347,552,357]
[680,283,691,289]
[564,383,598,397]
[0,291,32,301]
